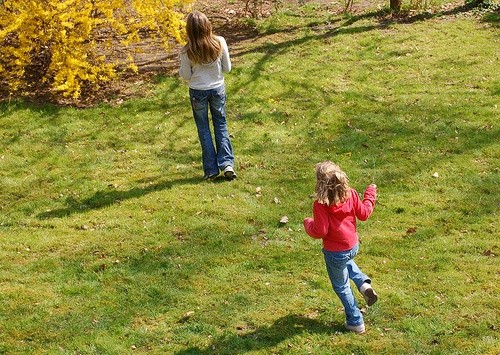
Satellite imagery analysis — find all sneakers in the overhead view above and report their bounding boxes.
[344,321,365,334]
[359,283,378,307]
[200,174,218,181]
[224,166,237,181]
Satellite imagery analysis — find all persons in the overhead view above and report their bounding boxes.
[303,161,378,335]
[180,9,238,181]
